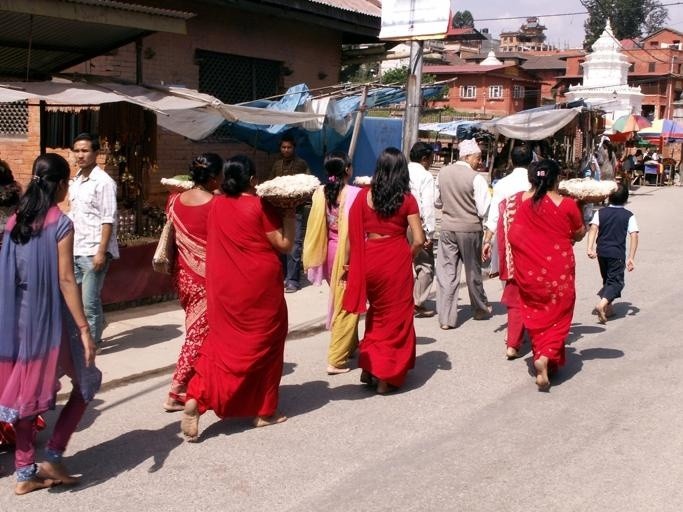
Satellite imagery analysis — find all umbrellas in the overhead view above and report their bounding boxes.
[611,113,683,144]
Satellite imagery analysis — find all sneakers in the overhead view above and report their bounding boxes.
[414,305,434,318]
[284,284,297,293]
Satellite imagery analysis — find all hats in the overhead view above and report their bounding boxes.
[458,137,482,159]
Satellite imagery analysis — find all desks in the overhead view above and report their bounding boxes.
[100,238,175,304]
[644,164,659,187]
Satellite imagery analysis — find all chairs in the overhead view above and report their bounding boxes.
[631,170,644,185]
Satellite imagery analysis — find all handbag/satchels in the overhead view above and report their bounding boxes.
[152,220,173,274]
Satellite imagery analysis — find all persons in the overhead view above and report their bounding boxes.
[434,138,493,331]
[271,138,310,292]
[303,152,362,373]
[165,153,227,410]
[623,147,660,179]
[1,153,102,494]
[525,139,619,223]
[407,141,436,317]
[181,156,297,441]
[70,138,119,346]
[344,147,424,394]
[481,147,586,390]
[586,186,639,321]
